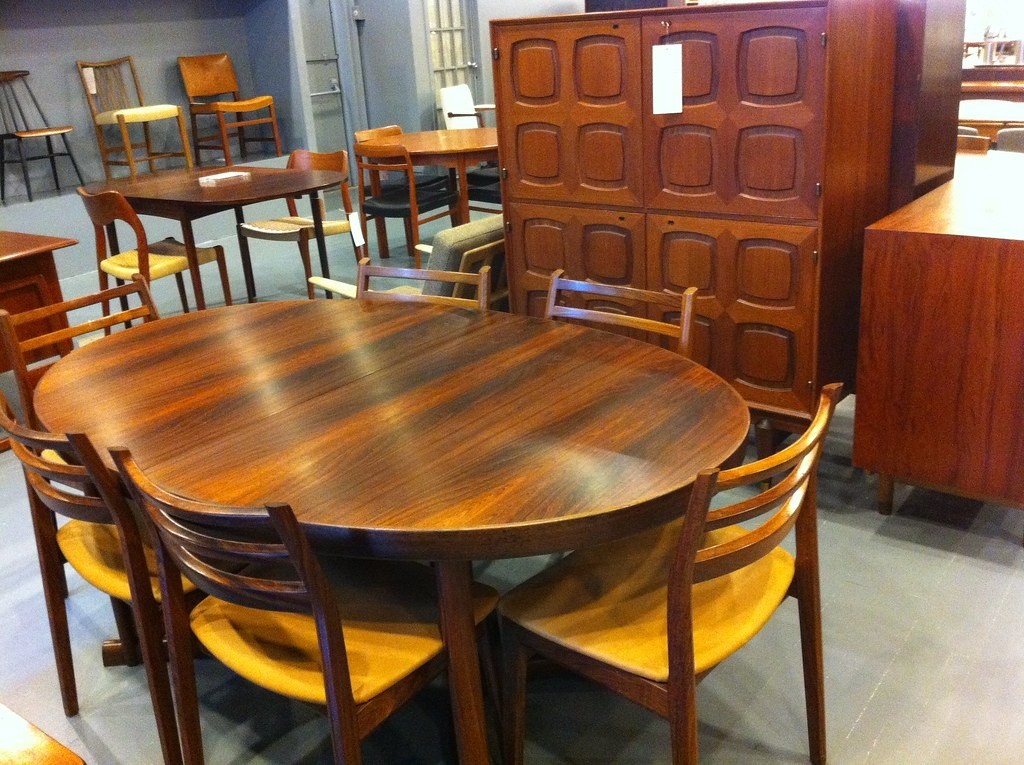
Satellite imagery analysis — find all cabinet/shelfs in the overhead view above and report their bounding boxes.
[1,229,80,451]
[489,1,1024,521]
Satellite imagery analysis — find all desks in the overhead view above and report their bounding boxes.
[365,128,499,258]
[30,302,750,764]
[83,165,348,329]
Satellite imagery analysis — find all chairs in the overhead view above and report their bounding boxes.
[1,387,277,765]
[0,70,85,202]
[236,148,371,303]
[76,185,232,336]
[177,53,282,167]
[105,447,499,765]
[309,126,512,312]
[0,273,161,600]
[497,381,844,765]
[76,57,193,180]
[544,268,699,360]
[439,84,496,130]
